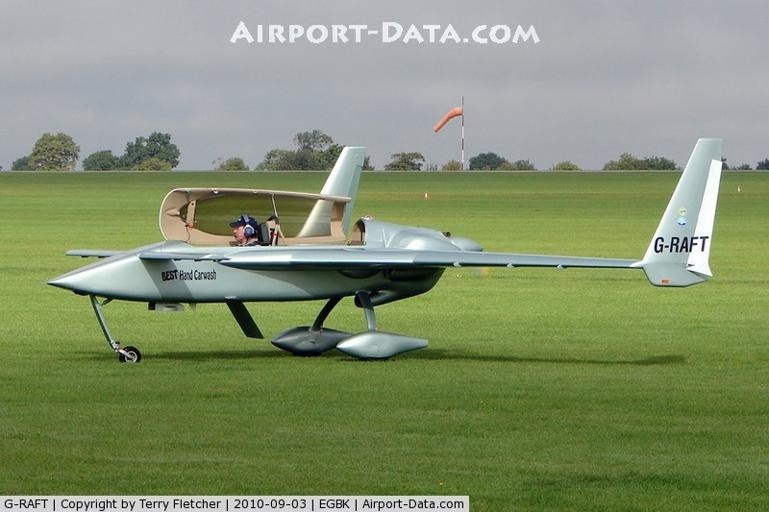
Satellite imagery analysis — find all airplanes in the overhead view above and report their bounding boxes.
[47,138,737,364]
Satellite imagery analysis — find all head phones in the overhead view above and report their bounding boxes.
[241,214,255,237]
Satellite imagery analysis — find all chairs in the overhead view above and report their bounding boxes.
[259,219,279,246]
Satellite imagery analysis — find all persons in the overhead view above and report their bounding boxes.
[229,217,263,247]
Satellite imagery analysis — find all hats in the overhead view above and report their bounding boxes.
[229,213,259,228]
